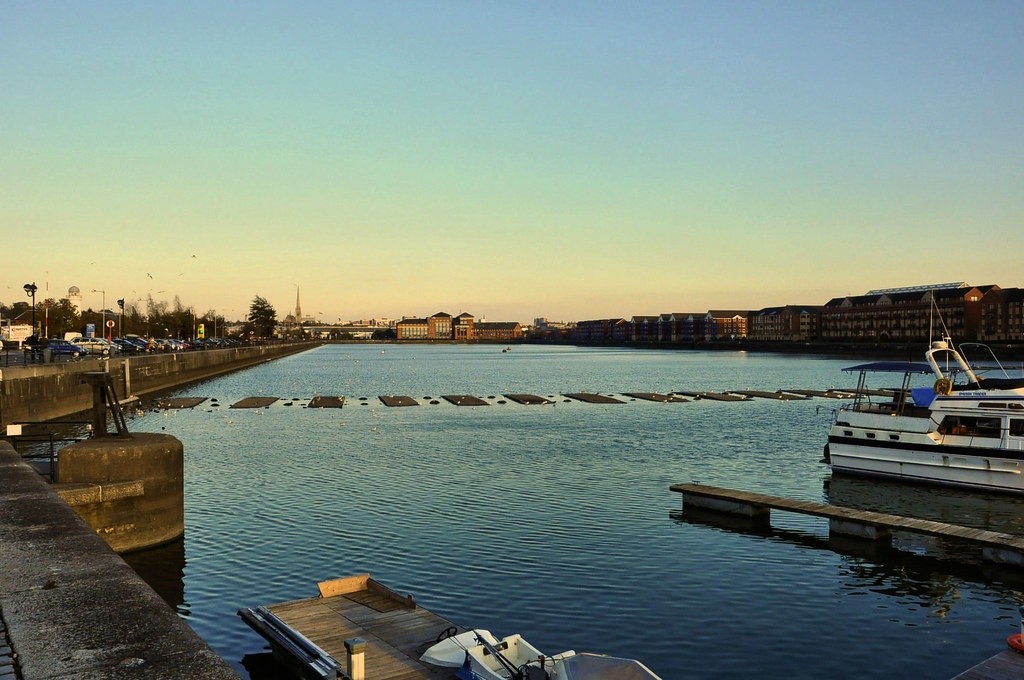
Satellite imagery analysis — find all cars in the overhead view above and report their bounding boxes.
[46,336,89,357]
[102,336,240,351]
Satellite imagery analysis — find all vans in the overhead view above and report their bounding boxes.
[69,337,121,355]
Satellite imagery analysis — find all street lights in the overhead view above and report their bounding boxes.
[91,288,105,339]
[117,298,124,337]
[24,282,37,364]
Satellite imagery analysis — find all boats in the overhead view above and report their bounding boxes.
[819,287,1024,496]
[236,572,661,680]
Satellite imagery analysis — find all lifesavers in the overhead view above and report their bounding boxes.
[933,379,953,397]
